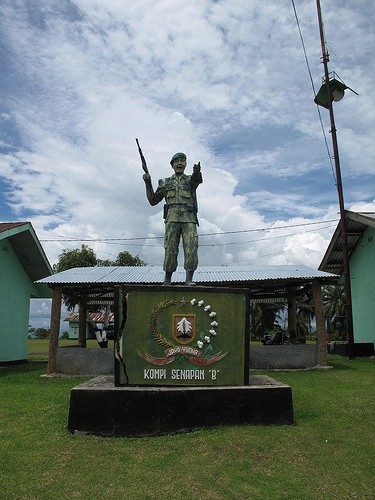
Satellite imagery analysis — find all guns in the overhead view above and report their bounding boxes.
[136,138,148,174]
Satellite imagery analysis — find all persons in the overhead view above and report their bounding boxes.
[143,153,203,286]
